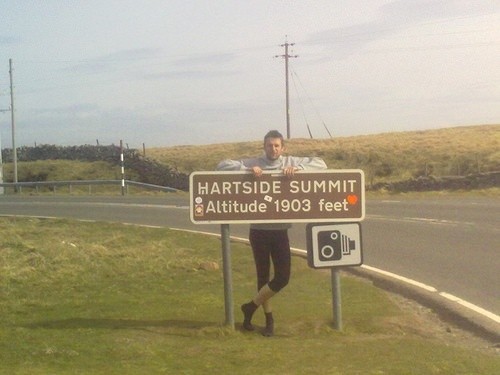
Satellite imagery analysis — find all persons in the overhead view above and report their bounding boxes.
[216,130,327,337]
